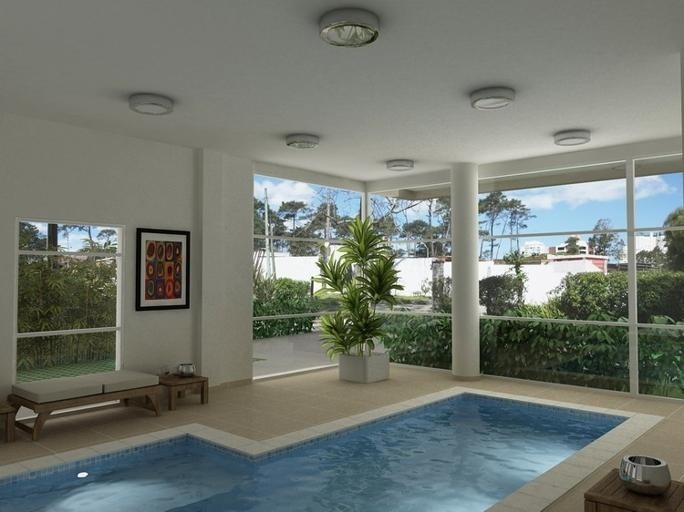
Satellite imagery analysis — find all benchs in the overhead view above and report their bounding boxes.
[8,369,162,441]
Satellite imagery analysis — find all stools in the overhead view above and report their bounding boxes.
[147,376,214,409]
[0,402,17,441]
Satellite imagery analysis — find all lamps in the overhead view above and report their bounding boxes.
[286,136,318,149]
[387,161,413,171]
[554,130,591,146]
[318,8,380,49]
[128,96,172,116]
[471,89,514,112]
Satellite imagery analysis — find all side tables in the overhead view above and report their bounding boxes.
[584,468,684,512]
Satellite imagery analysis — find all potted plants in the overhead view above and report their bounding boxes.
[314,213,404,385]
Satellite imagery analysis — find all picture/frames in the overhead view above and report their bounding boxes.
[135,227,190,312]
